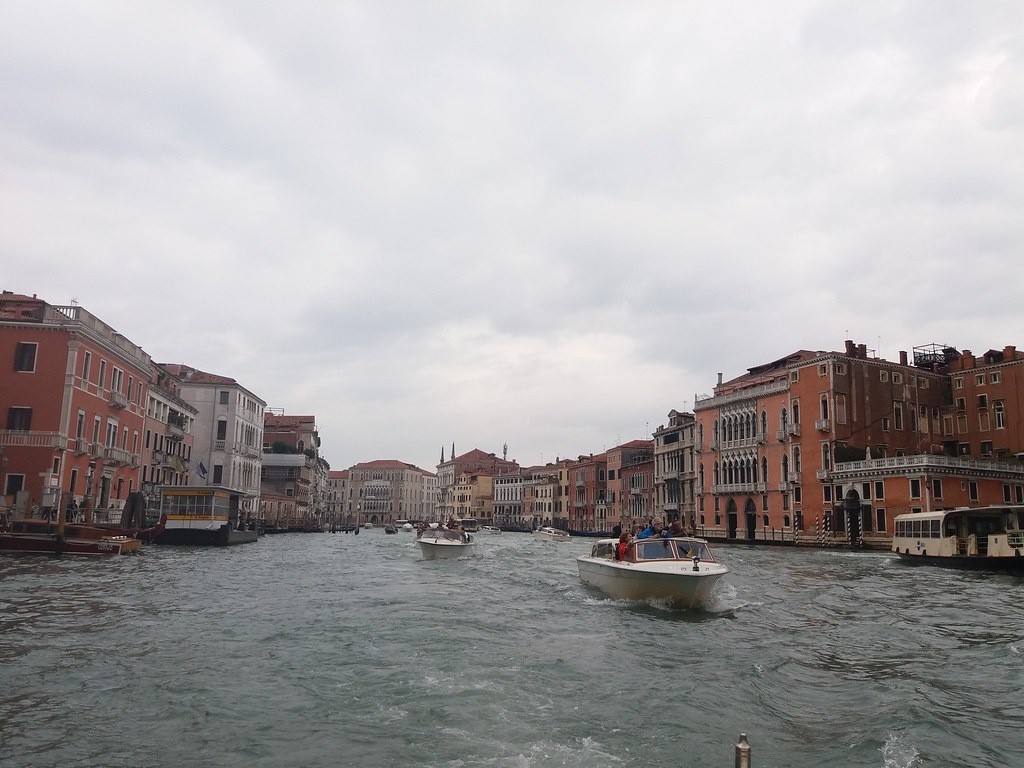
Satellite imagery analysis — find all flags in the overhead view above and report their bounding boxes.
[195,463,207,479]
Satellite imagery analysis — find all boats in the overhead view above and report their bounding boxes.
[477,524,501,535]
[890,503,1024,573]
[385,525,398,533]
[416,525,477,560]
[577,532,730,612]
[364,522,373,529]
[531,524,573,543]
[403,522,414,532]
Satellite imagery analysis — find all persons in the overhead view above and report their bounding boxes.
[519,519,548,532]
[611,516,703,562]
[417,517,470,544]
[31,495,122,524]
